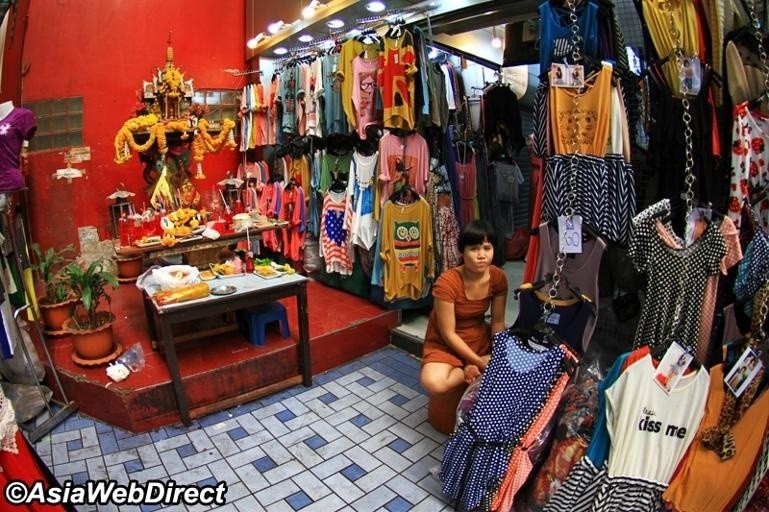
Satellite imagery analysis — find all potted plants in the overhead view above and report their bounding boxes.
[30,232,142,367]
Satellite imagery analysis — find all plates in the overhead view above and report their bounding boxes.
[252,264,289,280]
[210,284,237,296]
[209,262,246,279]
[198,270,216,281]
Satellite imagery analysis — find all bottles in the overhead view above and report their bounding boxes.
[155,213,165,236]
[119,218,129,246]
[246,242,255,273]
[223,198,243,226]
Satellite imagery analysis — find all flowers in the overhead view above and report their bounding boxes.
[142,215,312,427]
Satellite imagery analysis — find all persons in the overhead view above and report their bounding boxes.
[418,220,508,396]
[0,99,37,191]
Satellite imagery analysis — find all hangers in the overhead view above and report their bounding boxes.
[507,194,769,389]
[238,8,518,206]
[539,48,769,122]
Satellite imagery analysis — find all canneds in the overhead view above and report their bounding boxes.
[244,254,254,273]
